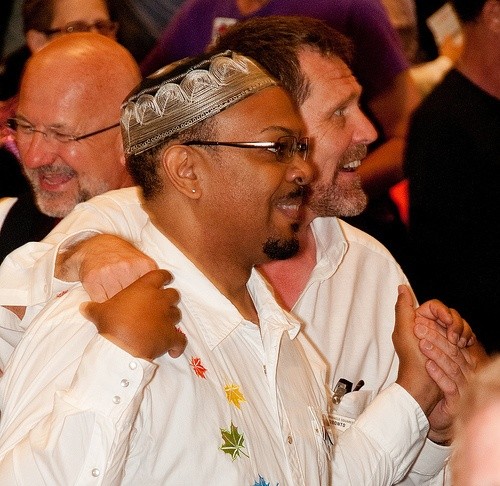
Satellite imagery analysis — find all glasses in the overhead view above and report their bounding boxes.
[7,118,120,149]
[181,136,309,164]
[47,20,119,38]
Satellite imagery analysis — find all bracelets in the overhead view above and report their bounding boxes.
[428,438,451,445]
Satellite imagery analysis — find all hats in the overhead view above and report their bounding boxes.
[120,50,277,158]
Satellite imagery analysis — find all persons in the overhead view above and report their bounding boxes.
[0,13,489,486]
[449,355,500,486]
[0,47,476,486]
[0,0,500,358]
